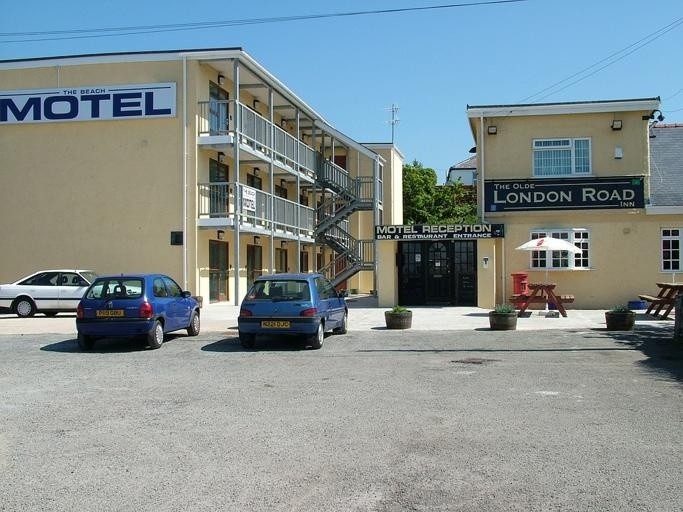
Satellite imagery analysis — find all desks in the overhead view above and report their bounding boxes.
[516,283,567,317]
[646,281,682,320]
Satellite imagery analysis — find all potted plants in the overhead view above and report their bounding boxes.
[384,304,412,329]
[488,302,517,330]
[603,303,636,330]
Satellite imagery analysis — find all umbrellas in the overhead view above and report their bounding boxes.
[514,235,583,311]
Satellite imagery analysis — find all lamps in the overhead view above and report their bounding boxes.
[487,125,497,136]
[611,119,623,130]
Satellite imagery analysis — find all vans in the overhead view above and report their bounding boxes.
[237,272,350,349]
[76,273,201,352]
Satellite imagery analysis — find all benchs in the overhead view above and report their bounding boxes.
[510,293,574,317]
[638,294,675,319]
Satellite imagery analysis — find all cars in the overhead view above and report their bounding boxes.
[0,269,142,319]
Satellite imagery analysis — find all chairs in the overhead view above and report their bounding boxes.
[36,278,53,284]
[268,286,283,298]
[61,275,78,285]
[113,286,126,298]
